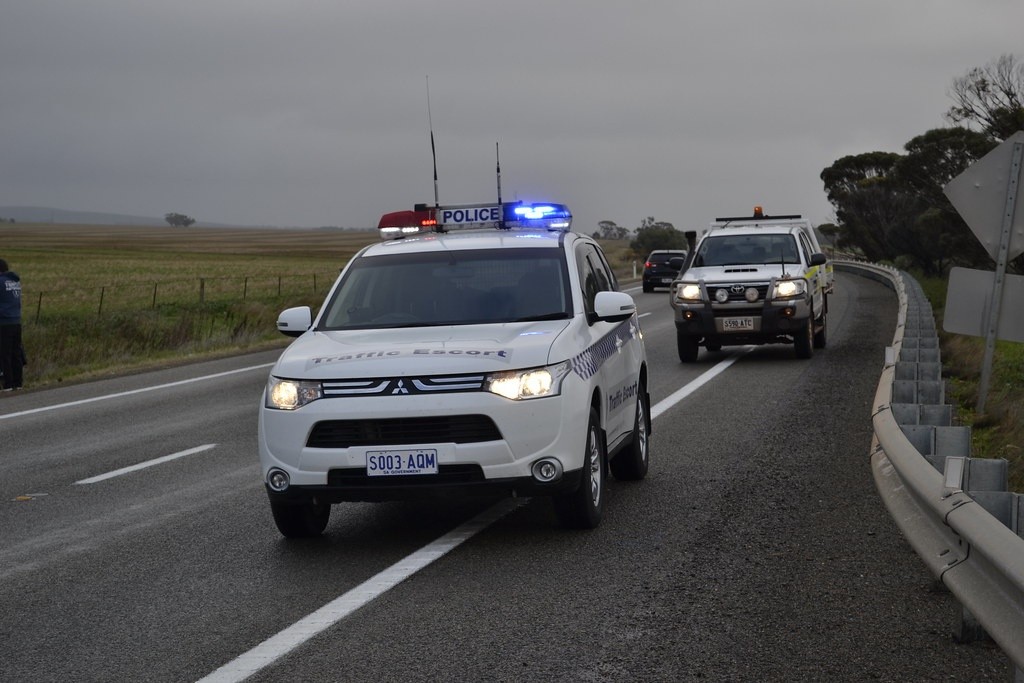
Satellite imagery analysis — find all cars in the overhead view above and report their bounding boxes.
[642,250,688,293]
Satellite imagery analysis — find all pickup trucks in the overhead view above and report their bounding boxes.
[669,206,834,361]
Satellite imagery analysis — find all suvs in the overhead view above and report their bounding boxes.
[254,198,651,541]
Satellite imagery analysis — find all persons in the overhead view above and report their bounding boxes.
[1,257,23,391]
